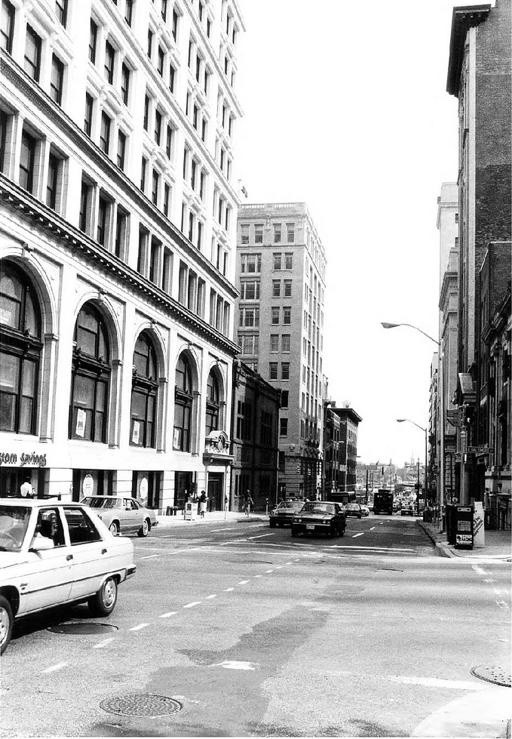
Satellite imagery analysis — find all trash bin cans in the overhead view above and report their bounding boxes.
[425,506,433,522]
[445,503,474,549]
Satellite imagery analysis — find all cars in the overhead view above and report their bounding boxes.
[0,496,137,656]
[269,488,425,541]
[50,494,160,538]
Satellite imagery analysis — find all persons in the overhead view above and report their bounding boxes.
[20,476,35,499]
[243,489,254,518]
[200,490,207,518]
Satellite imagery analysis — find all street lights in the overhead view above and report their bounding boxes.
[395,416,430,522]
[381,319,448,533]
